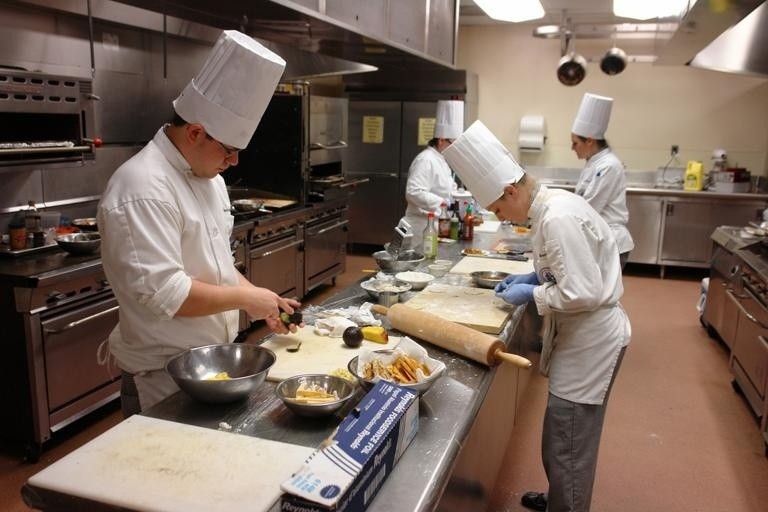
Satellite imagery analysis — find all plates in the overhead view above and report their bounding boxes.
[461,248,488,257]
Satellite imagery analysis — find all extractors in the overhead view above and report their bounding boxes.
[648,5,768,78]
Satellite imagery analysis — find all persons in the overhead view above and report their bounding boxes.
[486,171,632,512]
[571,134,635,272]
[404,138,457,247]
[96,114,304,419]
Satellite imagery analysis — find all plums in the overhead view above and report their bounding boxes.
[343,326,363,348]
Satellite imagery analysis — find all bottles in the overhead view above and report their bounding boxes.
[422,212,437,259]
[437,199,474,241]
[3,199,43,247]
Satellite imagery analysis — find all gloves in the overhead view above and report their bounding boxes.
[495,272,538,304]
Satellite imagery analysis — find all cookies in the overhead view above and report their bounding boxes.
[365,355,429,384]
[288,390,333,406]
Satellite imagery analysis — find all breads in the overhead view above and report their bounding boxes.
[360,326,388,344]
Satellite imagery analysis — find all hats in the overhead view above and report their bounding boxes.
[173,29,287,149]
[433,101,527,210]
[570,92,614,141]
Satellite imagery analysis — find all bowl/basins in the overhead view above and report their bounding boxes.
[274,373,356,415]
[372,249,426,271]
[165,344,277,401]
[358,277,411,297]
[232,198,263,214]
[71,216,99,230]
[472,271,513,288]
[55,232,102,255]
[396,270,434,289]
[429,258,452,277]
[348,350,445,395]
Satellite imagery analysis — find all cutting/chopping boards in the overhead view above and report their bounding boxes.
[399,281,515,333]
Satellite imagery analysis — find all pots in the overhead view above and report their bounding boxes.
[554,19,628,89]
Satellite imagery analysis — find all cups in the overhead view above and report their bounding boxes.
[39,212,61,233]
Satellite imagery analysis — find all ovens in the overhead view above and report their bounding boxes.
[231,208,311,341]
[24,261,122,442]
[240,94,370,290]
[722,285,767,400]
[0,66,100,166]
